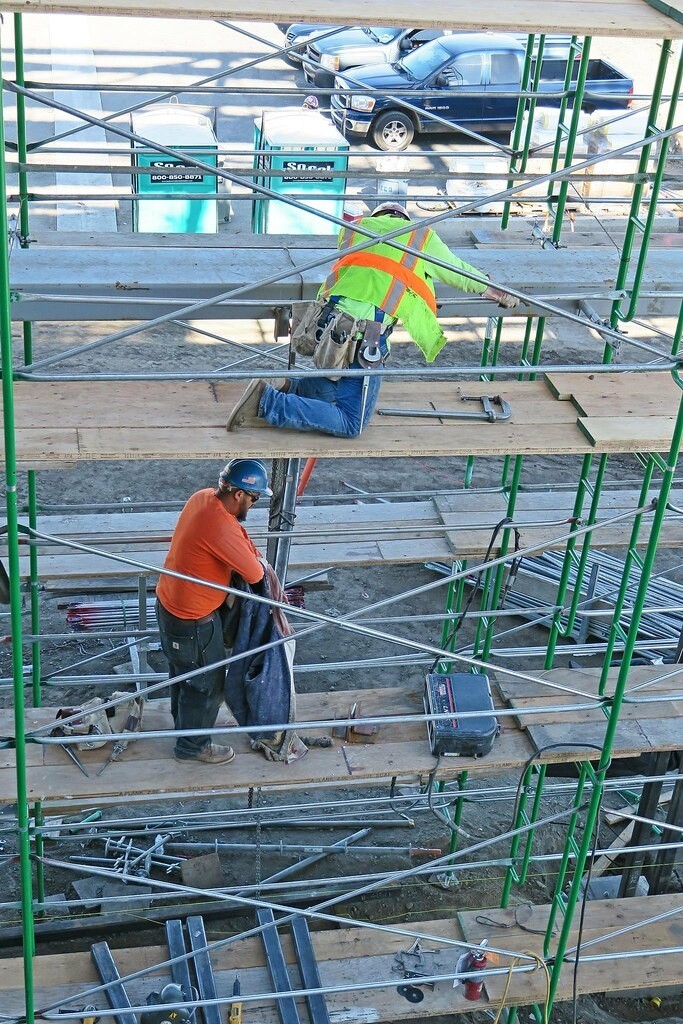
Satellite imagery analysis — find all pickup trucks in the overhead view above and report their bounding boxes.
[329,31,635,154]
[302,26,583,99]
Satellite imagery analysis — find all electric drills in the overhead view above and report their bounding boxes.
[229,973,243,1024]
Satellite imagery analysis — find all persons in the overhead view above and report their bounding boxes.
[227,199,531,440]
[154,459,273,767]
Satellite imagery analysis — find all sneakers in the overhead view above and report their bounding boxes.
[174,743,235,765]
[226,379,267,432]
[271,377,291,393]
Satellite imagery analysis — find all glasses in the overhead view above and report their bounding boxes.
[240,489,259,502]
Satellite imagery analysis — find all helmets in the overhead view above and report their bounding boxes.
[220,459,273,497]
[371,203,411,220]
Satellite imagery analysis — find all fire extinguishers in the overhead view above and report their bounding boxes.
[462,938,488,1000]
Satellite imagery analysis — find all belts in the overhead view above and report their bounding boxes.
[156,599,219,627]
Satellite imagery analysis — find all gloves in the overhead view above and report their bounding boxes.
[481,274,531,309]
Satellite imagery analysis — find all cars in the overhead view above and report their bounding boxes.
[283,24,346,63]
